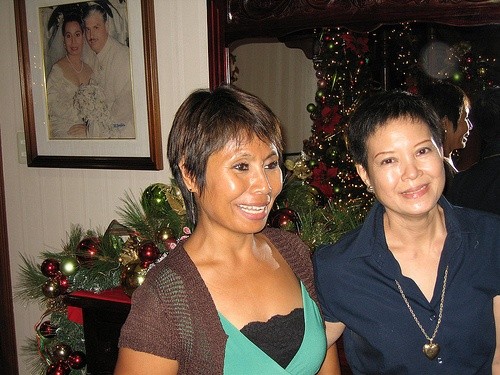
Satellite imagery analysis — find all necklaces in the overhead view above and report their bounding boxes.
[395,265,450,360]
[66,54,85,75]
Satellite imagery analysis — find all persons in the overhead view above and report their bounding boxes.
[64,1,136,138]
[313,88,500,375]
[423,84,473,207]
[111,83,341,375]
[47,3,96,138]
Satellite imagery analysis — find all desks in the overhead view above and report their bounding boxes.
[62,291,136,375]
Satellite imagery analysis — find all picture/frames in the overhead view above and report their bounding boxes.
[13,0,163,171]
